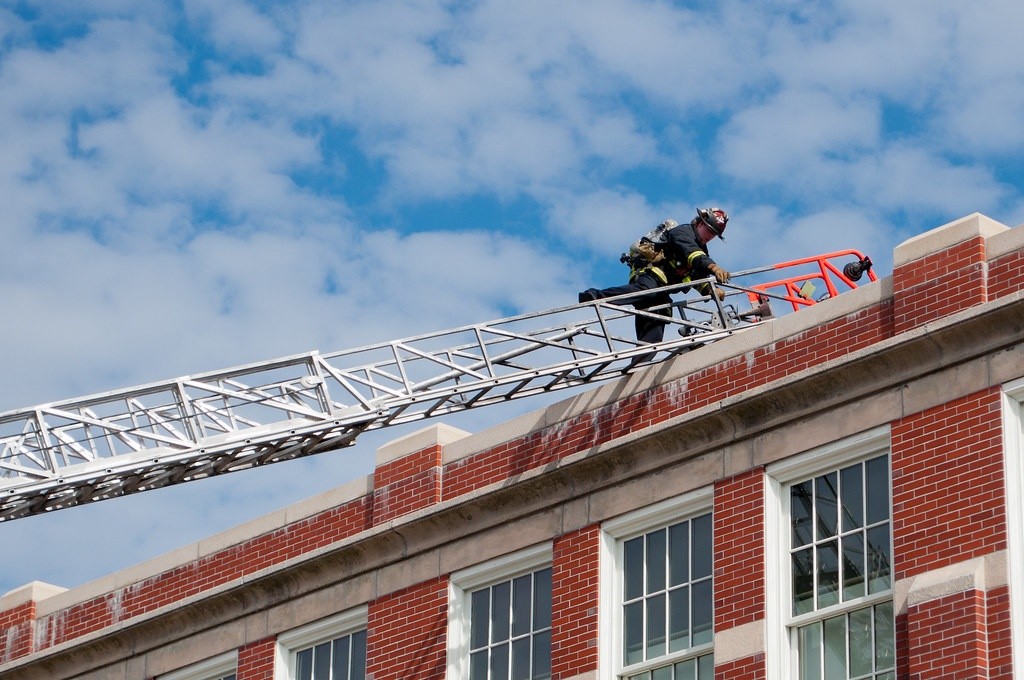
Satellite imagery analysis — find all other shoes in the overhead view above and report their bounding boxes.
[577,293,595,304]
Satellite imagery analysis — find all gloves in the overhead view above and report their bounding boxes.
[715,288,725,301]
[710,265,731,286]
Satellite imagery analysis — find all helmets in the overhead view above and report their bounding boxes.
[696,207,728,239]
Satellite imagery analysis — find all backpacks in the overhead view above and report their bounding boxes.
[620,219,680,265]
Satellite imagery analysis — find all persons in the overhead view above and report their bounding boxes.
[578,207,730,374]
